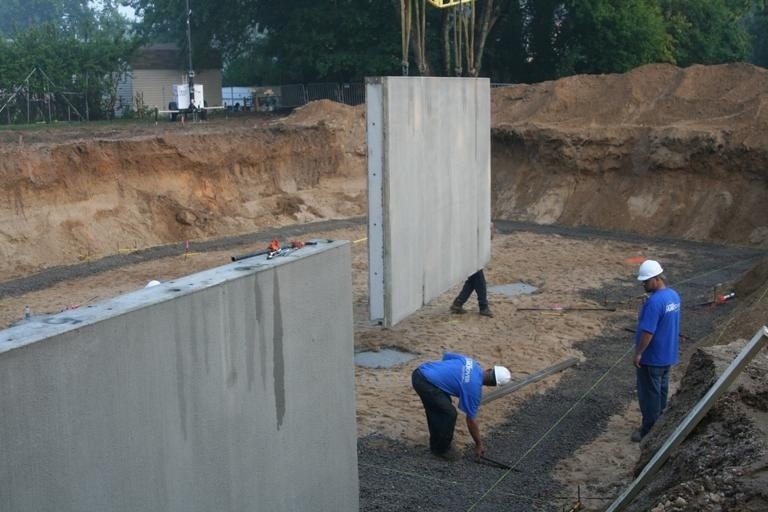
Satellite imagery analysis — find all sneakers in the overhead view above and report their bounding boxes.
[631,432,643,442]
[451,304,467,314]
[480,308,493,316]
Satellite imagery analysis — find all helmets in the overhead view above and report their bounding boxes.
[638,260,664,281]
[494,365,511,388]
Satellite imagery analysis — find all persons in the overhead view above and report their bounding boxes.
[631,259,681,442]
[449,223,497,318]
[411,351,511,459]
